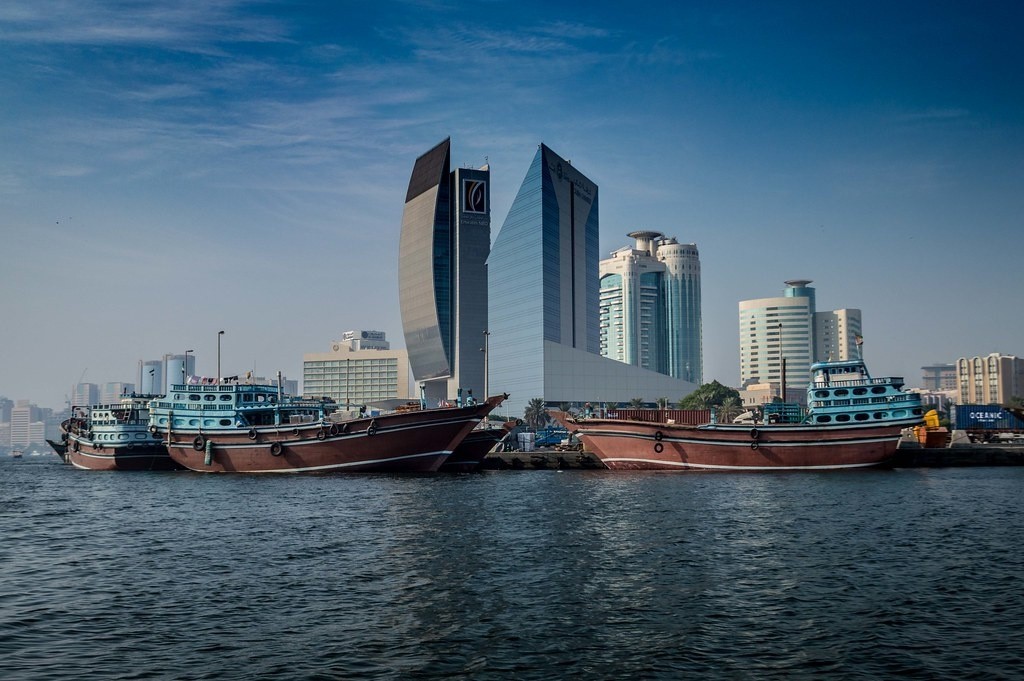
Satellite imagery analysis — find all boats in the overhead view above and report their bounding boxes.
[45,386,180,474]
[544,323,929,475]
[144,370,512,480]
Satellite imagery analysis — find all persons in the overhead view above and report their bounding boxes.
[753,408,761,425]
[64,412,125,439]
[585,403,593,419]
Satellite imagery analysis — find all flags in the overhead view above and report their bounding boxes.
[856,335,863,345]
[149,369,154,376]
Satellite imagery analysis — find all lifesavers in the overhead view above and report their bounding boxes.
[248,430,257,440]
[516,418,523,426]
[655,431,663,441]
[329,425,339,435]
[654,443,663,453]
[155,441,161,448]
[142,442,148,448]
[316,430,326,440]
[750,429,759,438]
[73,441,79,452]
[750,441,759,450]
[150,425,157,434]
[87,432,93,440]
[127,442,134,449]
[366,421,378,436]
[270,442,283,456]
[192,436,206,451]
[292,428,299,436]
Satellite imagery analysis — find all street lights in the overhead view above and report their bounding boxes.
[777,324,785,405]
[217,330,225,390]
[185,350,194,383]
[479,330,490,426]
